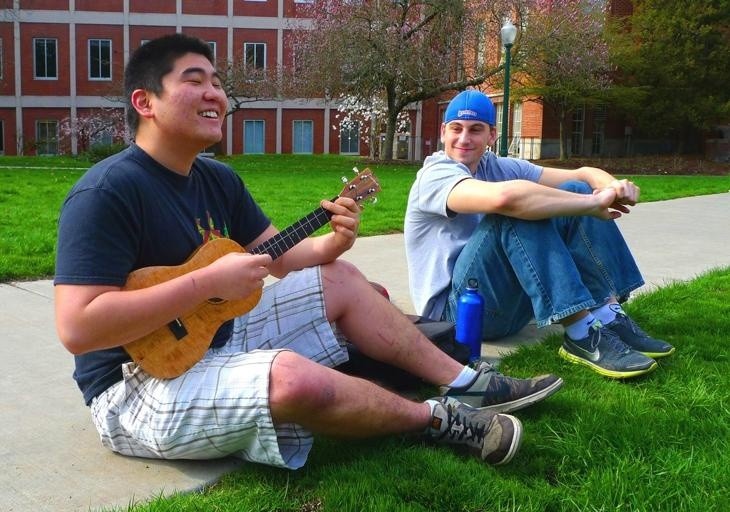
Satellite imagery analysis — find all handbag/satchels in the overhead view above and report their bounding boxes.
[336,312,469,390]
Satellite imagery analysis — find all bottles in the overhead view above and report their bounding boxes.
[453,278,486,364]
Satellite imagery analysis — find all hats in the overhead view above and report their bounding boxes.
[445,89,495,125]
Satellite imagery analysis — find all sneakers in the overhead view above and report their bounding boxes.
[597,314,676,357]
[420,395,522,466]
[558,326,658,380]
[439,358,563,416]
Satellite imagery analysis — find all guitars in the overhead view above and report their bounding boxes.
[116,165,382,378]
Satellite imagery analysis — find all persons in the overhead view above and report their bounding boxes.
[53,33,564,470]
[403,90,675,380]
